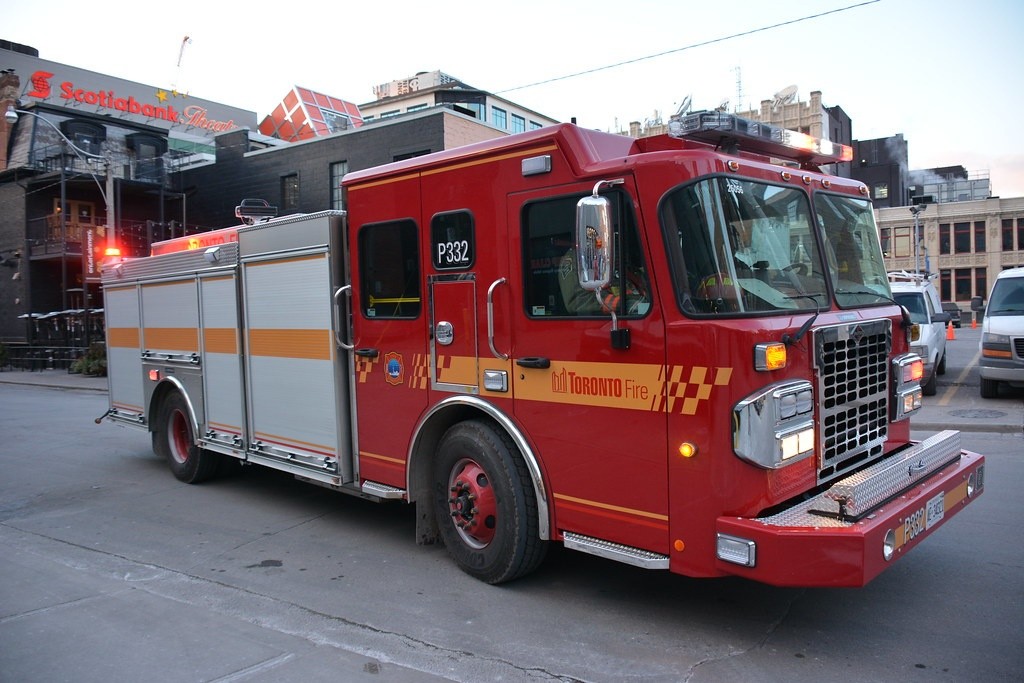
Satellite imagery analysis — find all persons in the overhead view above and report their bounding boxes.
[696,225,753,295]
[557,223,651,317]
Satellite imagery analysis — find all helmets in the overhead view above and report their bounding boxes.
[697,272,744,302]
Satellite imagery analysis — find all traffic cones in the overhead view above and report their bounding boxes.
[946,320,956,340]
[970,317,977,329]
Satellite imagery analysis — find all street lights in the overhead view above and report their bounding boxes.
[5,101,116,251]
[909,203,927,274]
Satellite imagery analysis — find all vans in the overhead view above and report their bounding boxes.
[971,268,1024,398]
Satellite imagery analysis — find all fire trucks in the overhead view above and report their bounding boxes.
[95,110,986,587]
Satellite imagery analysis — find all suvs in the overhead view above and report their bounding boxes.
[941,302,962,328]
[890,282,947,394]
